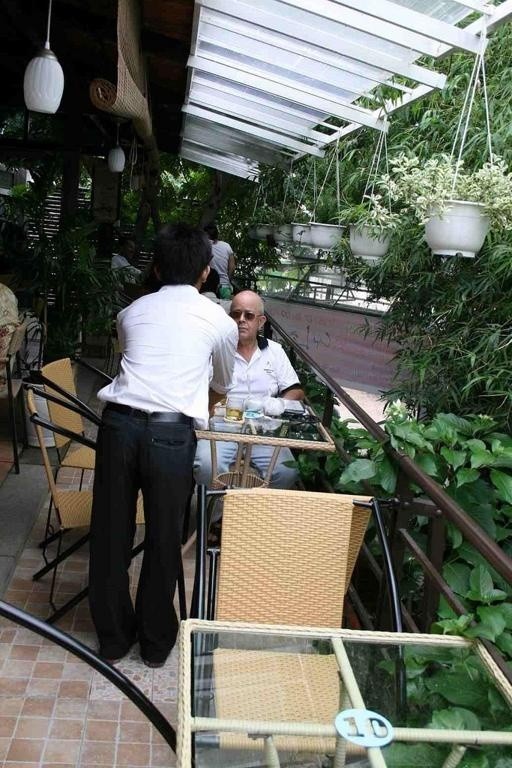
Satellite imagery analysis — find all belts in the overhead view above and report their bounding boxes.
[103,401,194,424]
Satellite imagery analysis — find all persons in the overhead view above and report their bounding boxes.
[111,240,143,300]
[91,221,239,668]
[200,224,236,299]
[0,283,20,380]
[199,266,233,314]
[193,290,306,548]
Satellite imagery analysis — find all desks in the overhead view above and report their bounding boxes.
[175,618,512,768]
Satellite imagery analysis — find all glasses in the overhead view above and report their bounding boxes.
[228,310,265,321]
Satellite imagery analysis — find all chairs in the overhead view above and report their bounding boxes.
[39,358,114,550]
[0,321,29,474]
[24,384,188,626]
[192,482,406,755]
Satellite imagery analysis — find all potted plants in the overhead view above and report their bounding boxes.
[361,19,512,259]
[330,114,403,261]
[308,129,347,251]
[291,158,318,248]
[242,163,292,245]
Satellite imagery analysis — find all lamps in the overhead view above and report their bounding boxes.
[23,1,66,114]
[108,117,128,173]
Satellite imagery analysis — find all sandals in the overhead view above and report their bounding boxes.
[207,518,222,547]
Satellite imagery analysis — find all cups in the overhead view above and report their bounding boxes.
[226,396,244,421]
[245,399,261,417]
